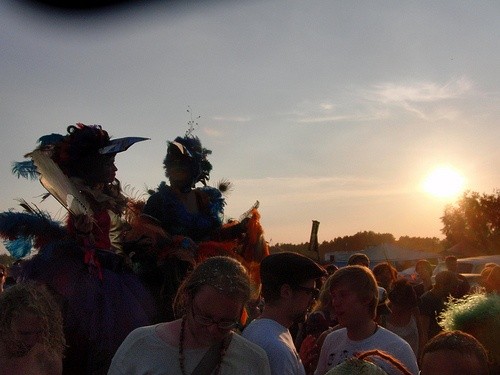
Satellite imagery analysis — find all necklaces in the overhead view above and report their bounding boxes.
[178,315,233,375]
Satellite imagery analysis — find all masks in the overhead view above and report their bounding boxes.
[338,297,374,328]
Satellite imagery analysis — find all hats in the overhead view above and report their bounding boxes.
[162,137,212,169]
[53,124,152,160]
[260,252,329,284]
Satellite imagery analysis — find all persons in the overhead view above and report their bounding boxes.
[106,257,271,375]
[0,279,70,375]
[142,136,270,336]
[0,254,32,294]
[421,330,489,375]
[240,251,328,375]
[289,253,500,375]
[313,264,420,375]
[326,349,414,375]
[0,125,163,375]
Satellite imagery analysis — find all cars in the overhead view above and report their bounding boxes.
[431,255,500,286]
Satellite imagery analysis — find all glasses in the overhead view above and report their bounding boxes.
[292,285,321,299]
[189,299,240,332]
